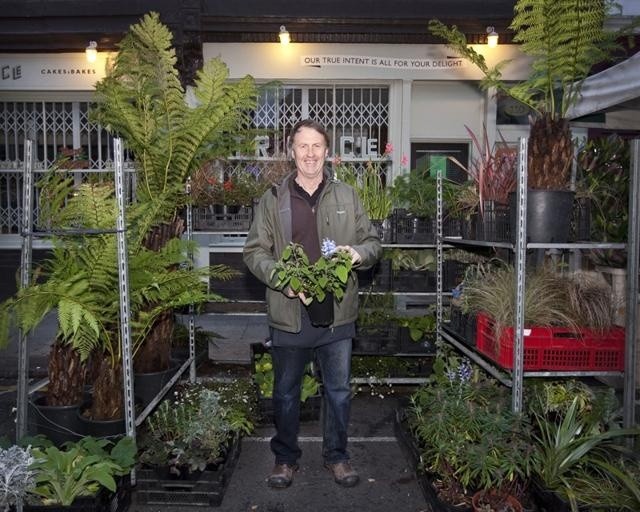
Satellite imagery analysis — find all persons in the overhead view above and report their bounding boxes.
[243,120,382,488]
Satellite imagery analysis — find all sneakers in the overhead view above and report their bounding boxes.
[268,462,297,490]
[327,460,360,487]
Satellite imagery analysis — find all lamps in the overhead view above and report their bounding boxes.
[85,40,99,62]
[487,25,499,50]
[278,25,291,48]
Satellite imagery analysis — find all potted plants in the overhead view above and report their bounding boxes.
[3,185,233,437]
[16,150,109,430]
[470,384,534,512]
[428,2,640,241]
[194,171,274,221]
[269,237,352,327]
[87,12,282,405]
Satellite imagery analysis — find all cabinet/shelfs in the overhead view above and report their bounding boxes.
[428,135,636,484]
[186,185,456,385]
